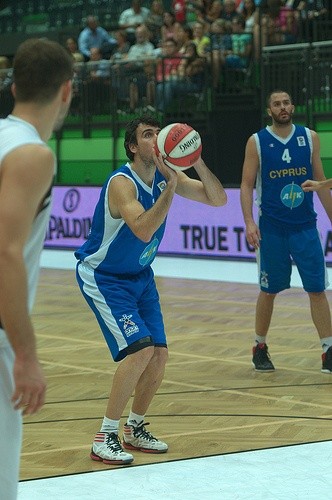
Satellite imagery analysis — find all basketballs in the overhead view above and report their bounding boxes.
[155,123,201,171]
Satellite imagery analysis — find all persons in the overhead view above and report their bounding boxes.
[0,0,332,119]
[301,178,332,193]
[301,177,332,193]
[240,89,332,374]
[0,38,75,500]
[73,116,228,465]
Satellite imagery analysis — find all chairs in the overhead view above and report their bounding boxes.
[0,0,332,130]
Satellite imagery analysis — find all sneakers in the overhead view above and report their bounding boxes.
[252,343,275,372]
[121,422,168,453]
[321,348,332,372]
[89,430,133,465]
